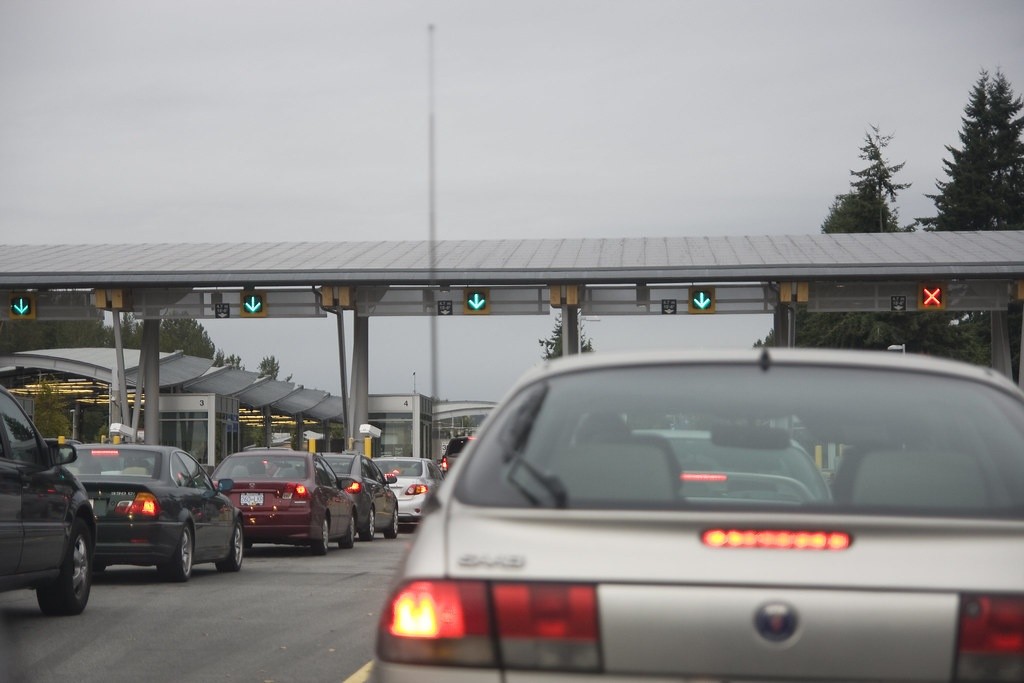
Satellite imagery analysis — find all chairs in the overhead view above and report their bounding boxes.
[122,467,150,476]
[851,450,990,507]
[551,434,683,504]
[231,466,250,476]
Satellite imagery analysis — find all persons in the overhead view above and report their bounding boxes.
[576,415,690,509]
[248,460,266,477]
